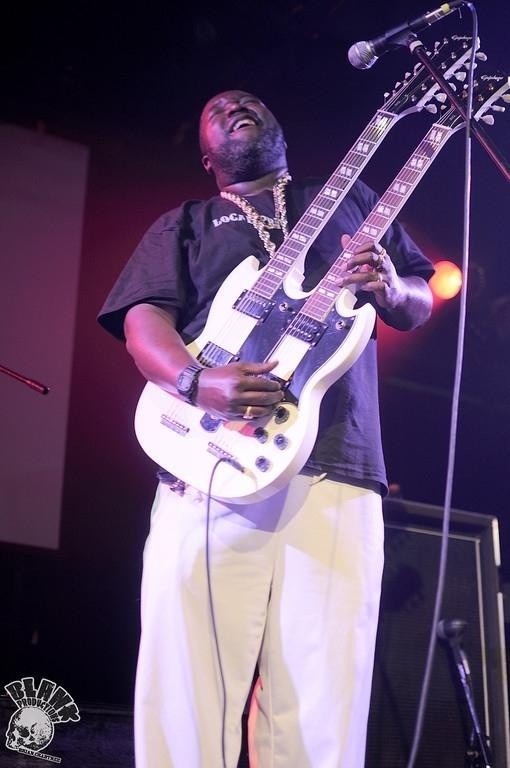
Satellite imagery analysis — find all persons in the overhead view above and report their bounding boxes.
[94,88,437,767]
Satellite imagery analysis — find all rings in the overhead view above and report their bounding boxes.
[244,405,254,421]
[376,250,386,283]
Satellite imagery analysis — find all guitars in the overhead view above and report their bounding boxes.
[133,33,510,507]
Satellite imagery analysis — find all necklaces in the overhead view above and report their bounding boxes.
[220,171,293,261]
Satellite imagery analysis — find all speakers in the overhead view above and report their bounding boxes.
[0,538,117,703]
[361,496,509,768]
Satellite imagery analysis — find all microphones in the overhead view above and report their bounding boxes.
[433,618,467,637]
[346,0,465,70]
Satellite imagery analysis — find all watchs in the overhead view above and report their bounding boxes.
[176,363,202,405]
